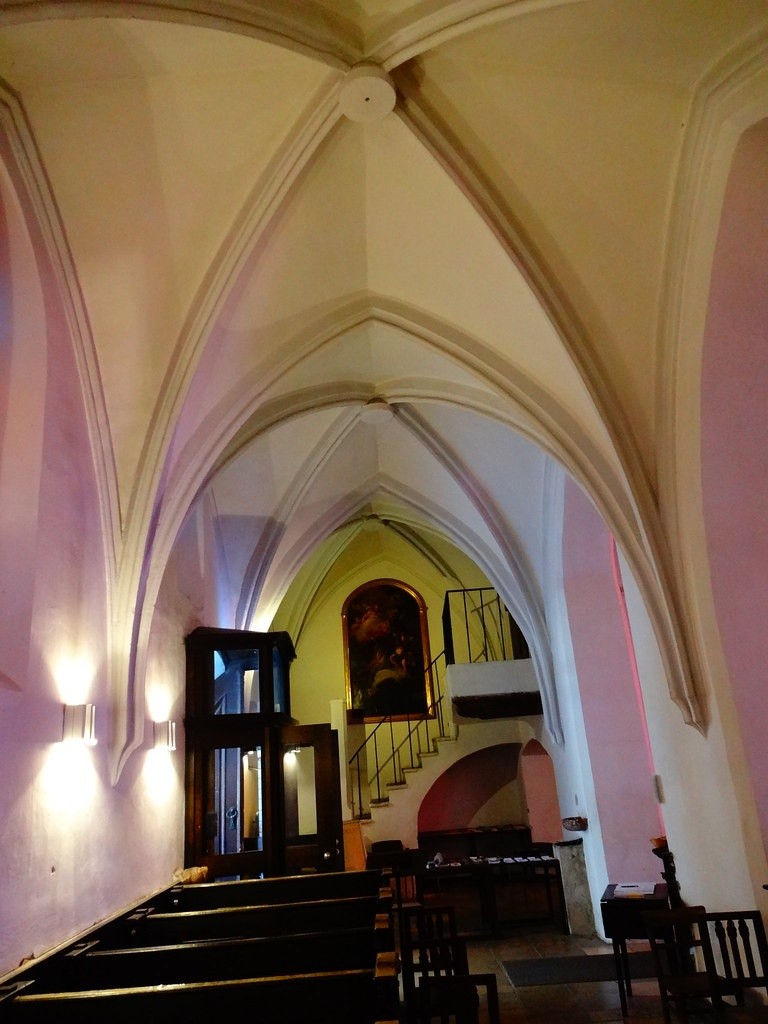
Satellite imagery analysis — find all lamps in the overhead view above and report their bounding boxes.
[61,704,96,741]
[153,720,177,751]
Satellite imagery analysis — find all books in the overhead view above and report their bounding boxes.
[614,882,656,895]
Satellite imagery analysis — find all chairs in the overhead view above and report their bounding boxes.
[639,904,768,1024]
[398,905,501,1024]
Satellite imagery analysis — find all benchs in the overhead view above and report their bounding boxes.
[0,866,405,1024]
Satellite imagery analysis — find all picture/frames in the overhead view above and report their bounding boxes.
[342,577,437,725]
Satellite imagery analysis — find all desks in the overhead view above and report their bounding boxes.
[417,859,566,935]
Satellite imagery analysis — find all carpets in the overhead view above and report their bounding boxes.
[503,950,658,989]
